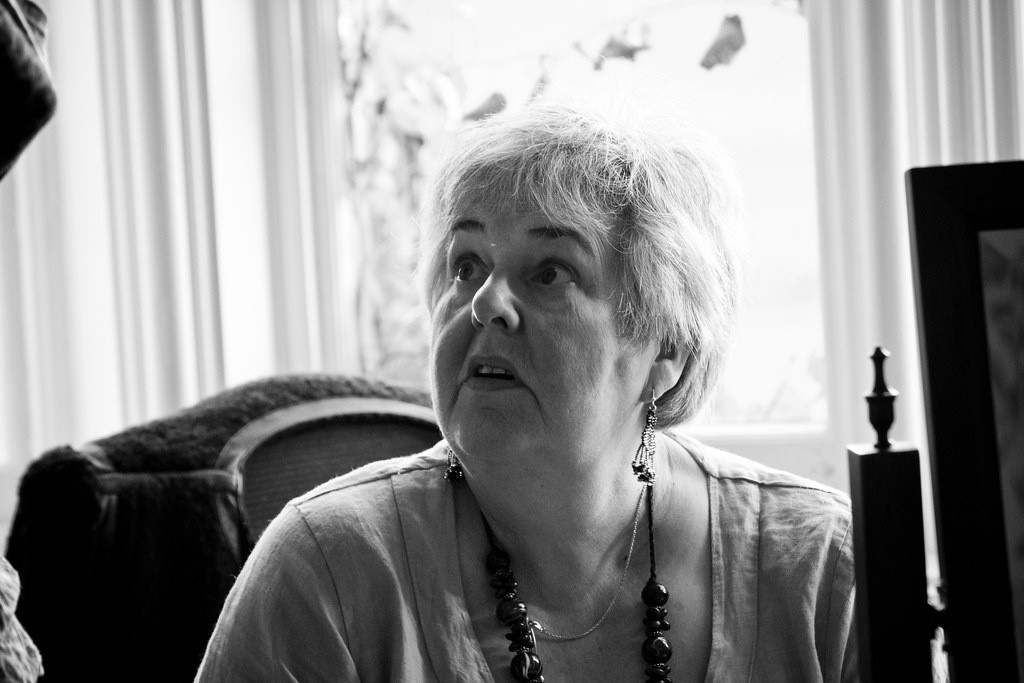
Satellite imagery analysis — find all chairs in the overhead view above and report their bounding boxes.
[6,375,444,683]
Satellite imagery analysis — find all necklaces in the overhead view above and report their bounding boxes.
[484,485,674,683]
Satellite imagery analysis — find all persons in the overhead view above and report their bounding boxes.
[191,112,947,683]
